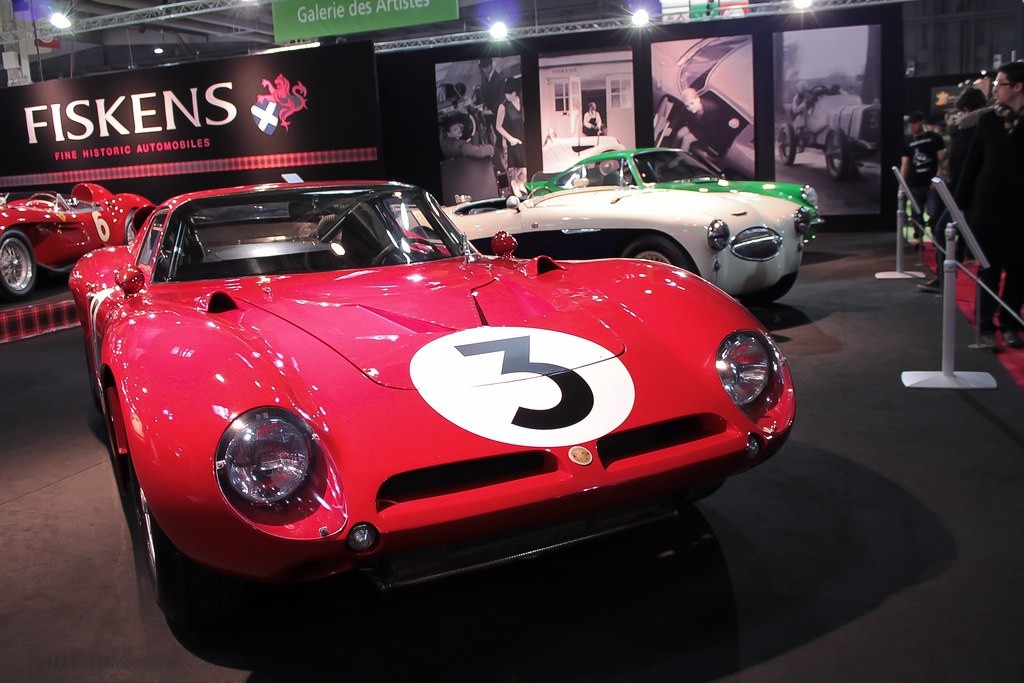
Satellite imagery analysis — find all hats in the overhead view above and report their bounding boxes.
[907,110,927,122]
[477,58,492,65]
[441,116,459,130]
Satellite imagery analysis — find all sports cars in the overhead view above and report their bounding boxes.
[0,180,157,306]
[523,146,826,251]
[392,179,810,319]
[65,179,799,633]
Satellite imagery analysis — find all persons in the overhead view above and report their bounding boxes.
[583,102,603,136]
[441,58,529,199]
[928,86,989,295]
[793,80,811,145]
[676,87,740,158]
[957,63,1023,335]
[899,113,941,242]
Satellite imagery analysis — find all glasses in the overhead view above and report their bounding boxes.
[993,80,1012,87]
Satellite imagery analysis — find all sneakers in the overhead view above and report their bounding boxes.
[979,331,1003,353]
[1003,328,1024,348]
[917,276,945,293]
